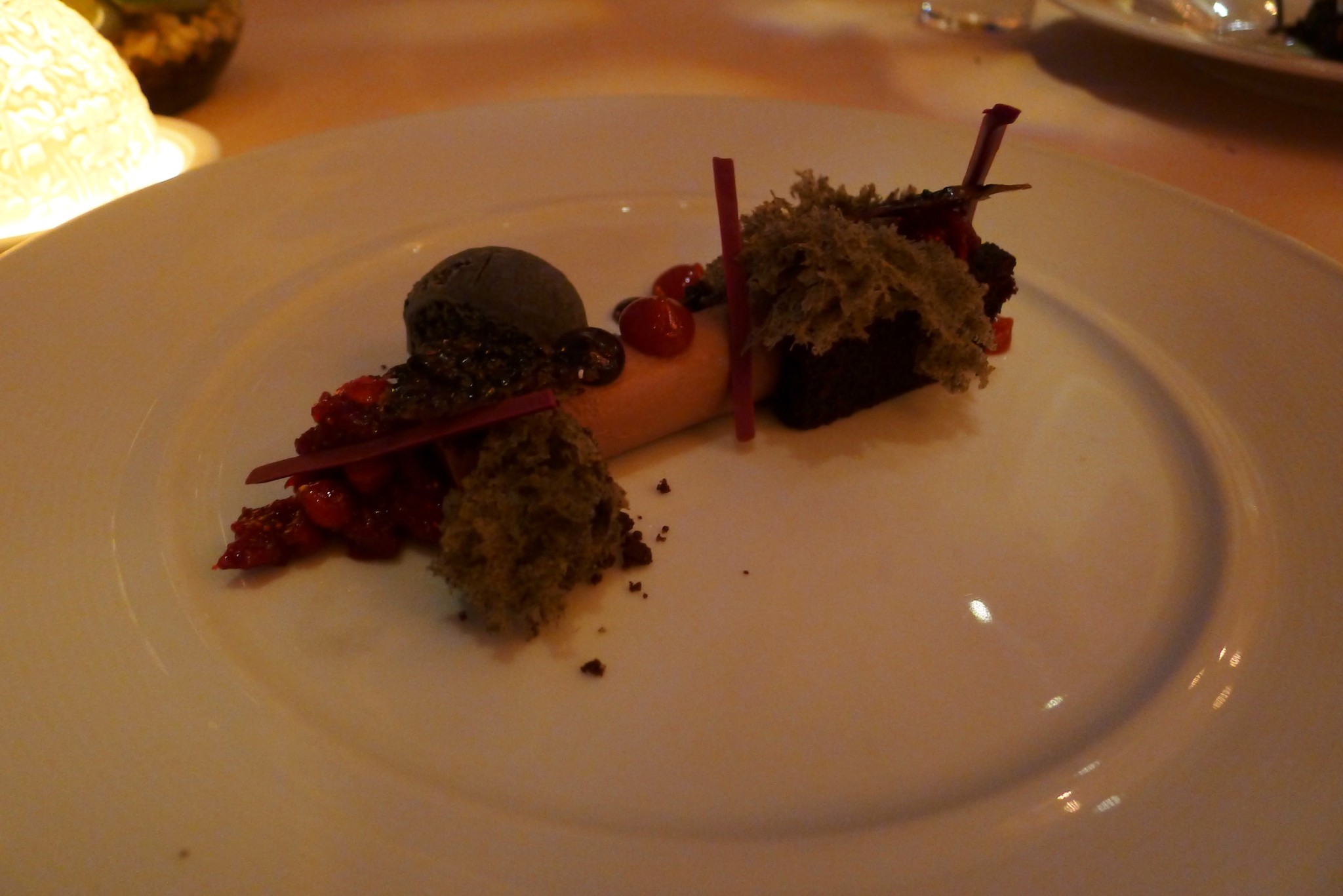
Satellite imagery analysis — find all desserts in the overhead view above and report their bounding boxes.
[209,101,1032,636]
[52,0,242,117]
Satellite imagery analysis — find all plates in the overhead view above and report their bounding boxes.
[1054,0,1342,81]
[0,93,1342,896]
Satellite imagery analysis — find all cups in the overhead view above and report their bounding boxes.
[919,0,1035,40]
